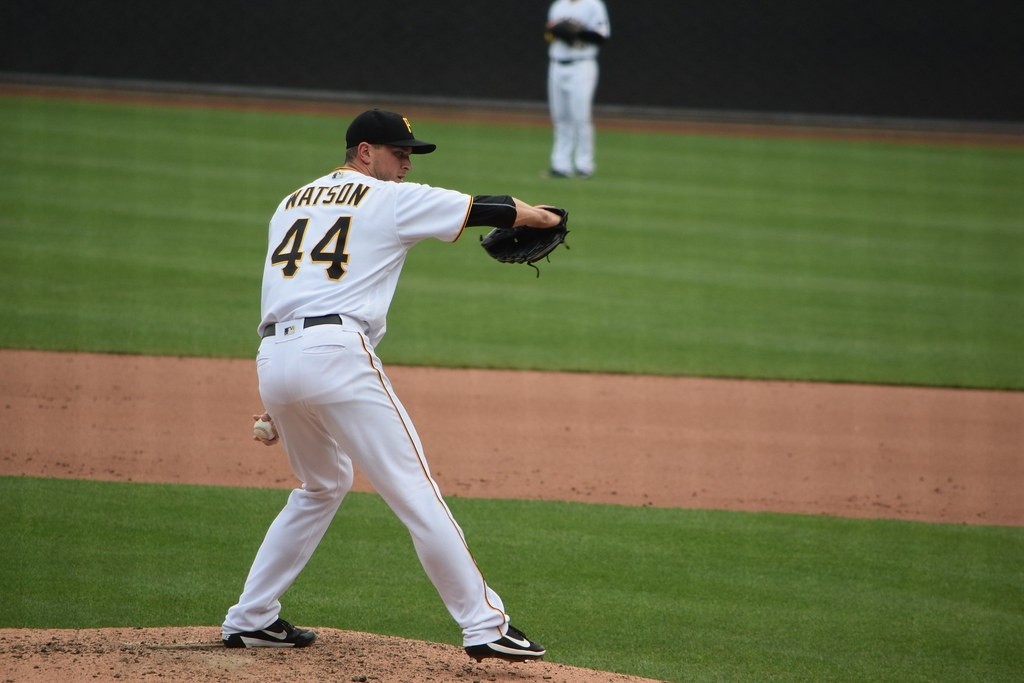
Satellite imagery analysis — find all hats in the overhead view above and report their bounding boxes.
[346,108,437,154]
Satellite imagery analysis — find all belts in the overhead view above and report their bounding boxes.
[261,315,342,336]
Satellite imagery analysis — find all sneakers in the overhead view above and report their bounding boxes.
[465,624,547,664]
[222,617,317,648]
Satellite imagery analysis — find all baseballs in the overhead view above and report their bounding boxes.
[253,415,276,441]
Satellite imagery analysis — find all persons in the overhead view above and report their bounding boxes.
[544,0,610,178]
[221,108,570,663]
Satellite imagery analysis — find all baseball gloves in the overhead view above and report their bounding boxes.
[479,208,572,265]
